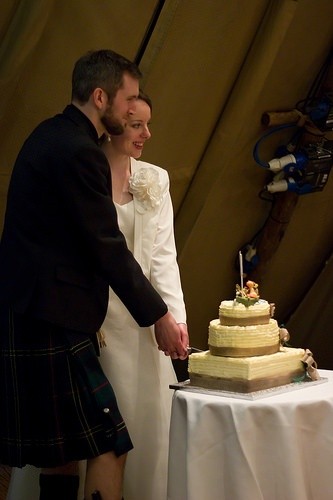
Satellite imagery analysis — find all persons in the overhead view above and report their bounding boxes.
[0,49,187,500]
[8,88,190,500]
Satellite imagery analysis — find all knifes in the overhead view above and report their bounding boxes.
[158,346,202,356]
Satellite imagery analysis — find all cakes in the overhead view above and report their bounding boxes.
[187,299,306,393]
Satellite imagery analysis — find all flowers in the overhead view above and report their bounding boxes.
[128,168,162,211]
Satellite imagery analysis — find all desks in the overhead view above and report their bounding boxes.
[166,367,333,500]
